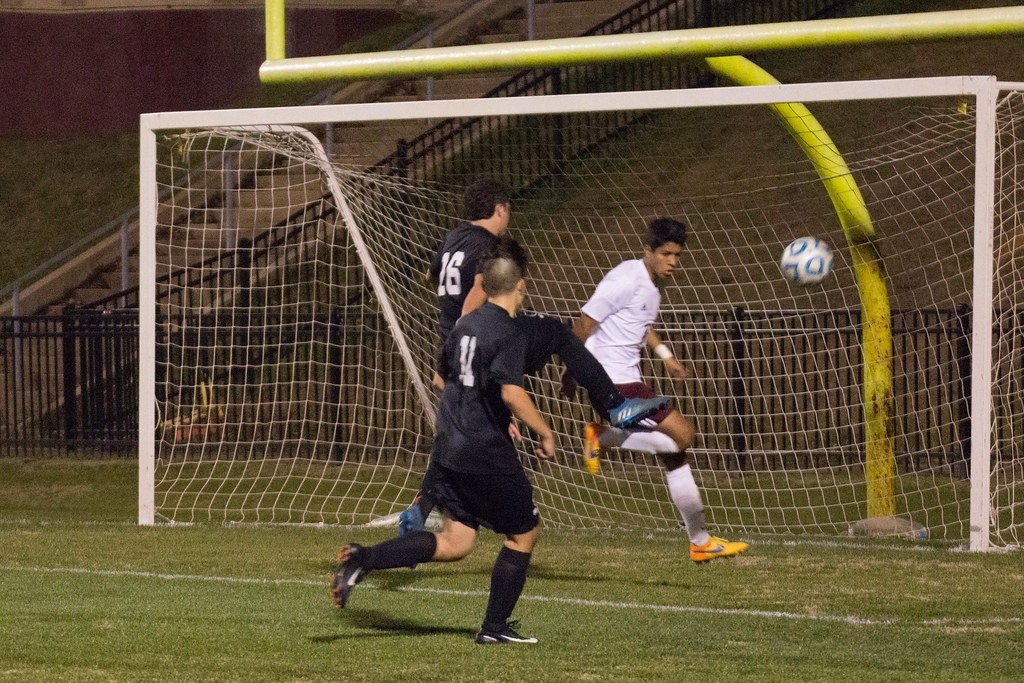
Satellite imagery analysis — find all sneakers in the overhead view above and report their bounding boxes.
[330,543,368,610]
[475,620,538,644]
[583,421,601,475]
[607,397,671,429]
[690,535,750,561]
[398,505,424,569]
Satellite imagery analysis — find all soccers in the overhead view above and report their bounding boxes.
[780,235,834,287]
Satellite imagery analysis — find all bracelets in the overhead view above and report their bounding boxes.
[652,344,675,363]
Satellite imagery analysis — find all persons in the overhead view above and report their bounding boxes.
[329,238,555,646]
[560,216,752,562]
[397,175,670,570]
